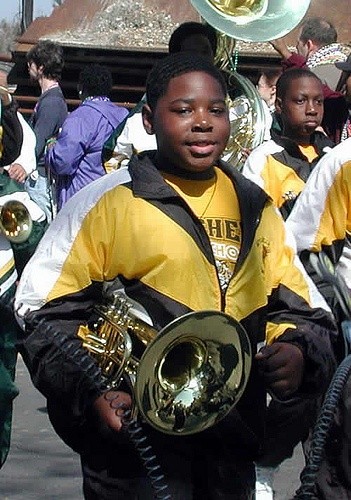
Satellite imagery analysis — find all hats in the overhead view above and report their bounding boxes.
[335,52,351,72]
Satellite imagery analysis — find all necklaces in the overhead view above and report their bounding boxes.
[198,166,218,219]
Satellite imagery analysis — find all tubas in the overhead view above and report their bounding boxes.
[189,0,311,172]
[82,291,252,436]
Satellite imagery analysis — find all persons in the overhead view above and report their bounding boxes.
[12,52,342,500]
[0,166,47,467]
[26,40,68,223]
[256,70,286,113]
[46,64,131,214]
[7,50,31,84]
[335,52,351,142]
[286,135,351,500]
[269,17,351,142]
[1,85,37,184]
[101,22,254,175]
[241,68,337,222]
[0,85,24,168]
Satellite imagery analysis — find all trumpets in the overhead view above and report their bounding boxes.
[0,200,32,242]
